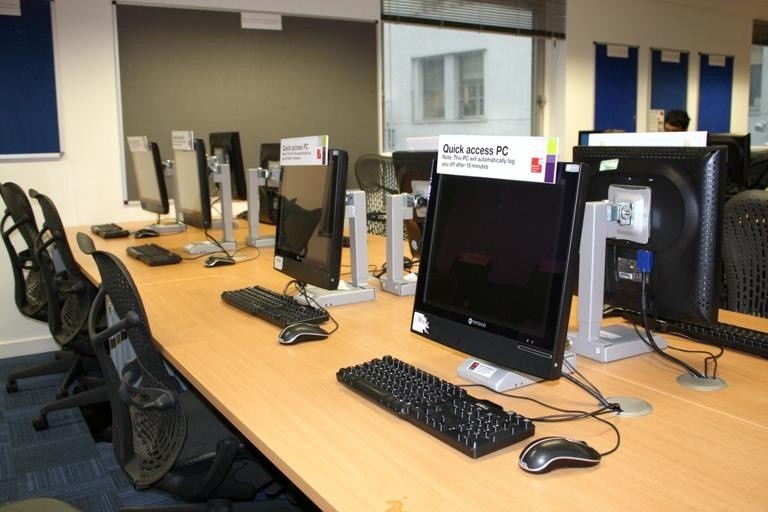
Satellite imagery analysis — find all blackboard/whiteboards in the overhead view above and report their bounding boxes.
[0,1,66,161]
[112,0,385,206]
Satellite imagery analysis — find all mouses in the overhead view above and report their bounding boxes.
[518,436,600,474]
[134,229,158,239]
[382,256,412,271]
[277,324,328,345]
[203,256,235,268]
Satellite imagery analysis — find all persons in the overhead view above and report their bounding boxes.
[662,110,691,131]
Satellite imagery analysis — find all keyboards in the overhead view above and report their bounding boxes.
[90,222,129,239]
[126,243,181,267]
[343,235,350,248]
[623,312,768,359]
[221,285,328,330]
[336,354,535,458]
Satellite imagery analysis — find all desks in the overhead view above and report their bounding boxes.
[45,217,420,291]
[136,250,767,511]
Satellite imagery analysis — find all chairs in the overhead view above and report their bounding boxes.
[0,180,102,402]
[27,185,115,446]
[716,188,768,321]
[352,152,415,243]
[76,232,307,512]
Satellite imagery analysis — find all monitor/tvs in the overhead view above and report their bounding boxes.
[171,138,237,255]
[131,141,187,233]
[578,131,604,146]
[245,143,280,249]
[410,155,590,396]
[208,132,246,229]
[274,148,375,310]
[566,146,726,364]
[706,133,750,188]
[380,151,438,296]
